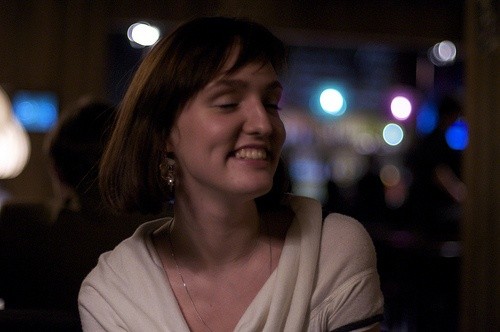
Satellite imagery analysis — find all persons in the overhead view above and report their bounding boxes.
[78,17,384,332]
[0,98,137,332]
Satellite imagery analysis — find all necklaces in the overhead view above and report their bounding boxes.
[168,218,272,332]
